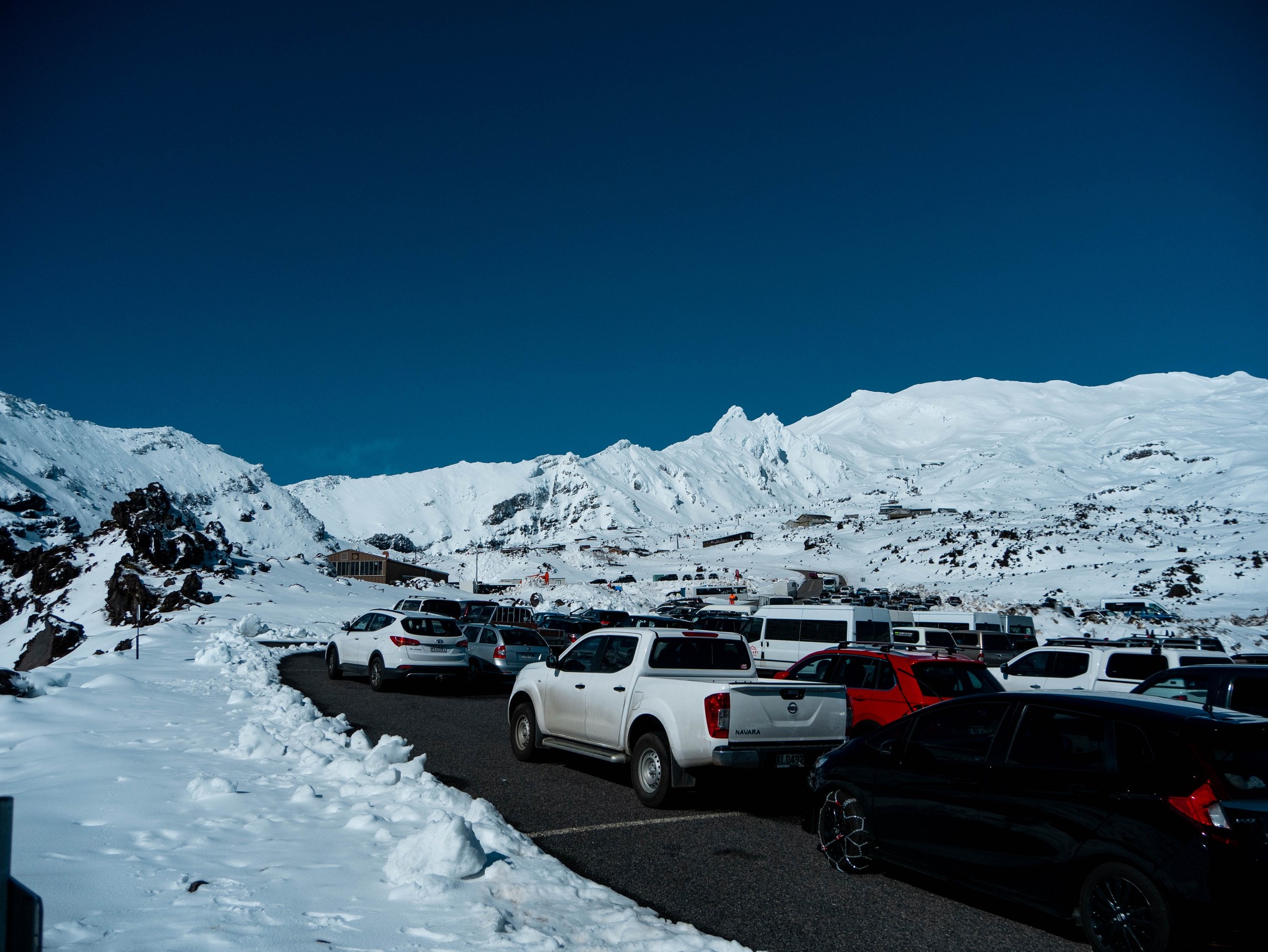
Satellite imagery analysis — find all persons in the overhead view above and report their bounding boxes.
[729,593,735,604]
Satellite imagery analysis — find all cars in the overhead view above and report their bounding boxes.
[383,576,1227,678]
[458,623,553,689]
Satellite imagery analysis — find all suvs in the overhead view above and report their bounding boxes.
[773,638,1007,742]
[1128,664,1268,718]
[805,684,1268,952]
[324,608,469,692]
[954,643,1237,703]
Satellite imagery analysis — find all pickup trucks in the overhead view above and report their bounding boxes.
[507,627,852,807]
